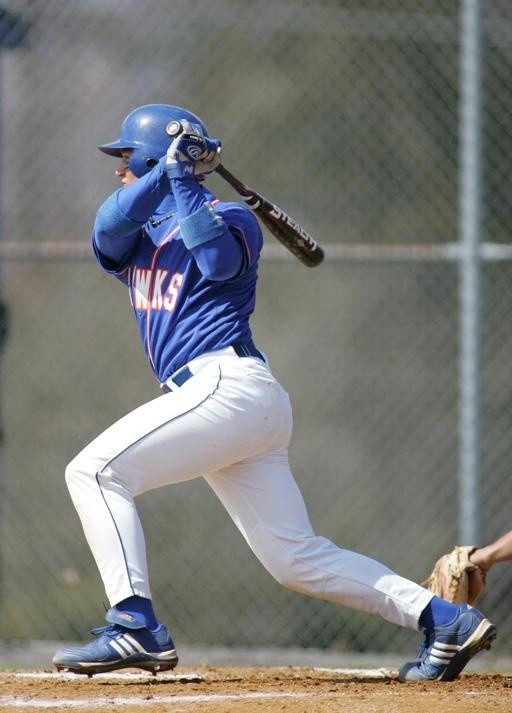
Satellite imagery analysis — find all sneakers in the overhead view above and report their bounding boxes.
[53,607,178,678]
[399,546,497,684]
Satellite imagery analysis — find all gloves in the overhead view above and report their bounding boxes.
[159,118,222,179]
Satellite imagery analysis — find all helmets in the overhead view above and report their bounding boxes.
[97,104,207,181]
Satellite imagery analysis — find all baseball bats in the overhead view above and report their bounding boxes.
[165,120,324,268]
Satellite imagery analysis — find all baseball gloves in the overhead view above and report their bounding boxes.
[421,546,487,608]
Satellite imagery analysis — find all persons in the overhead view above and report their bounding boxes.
[51,102,499,682]
[418,527,511,606]
